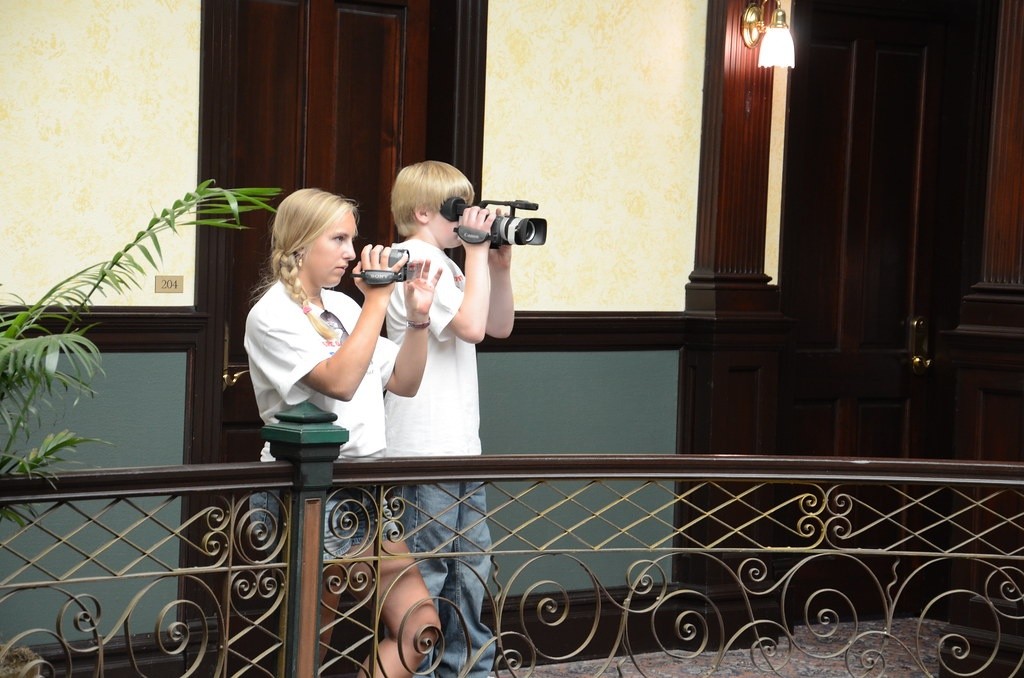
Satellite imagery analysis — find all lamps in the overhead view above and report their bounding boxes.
[741,0,797,71]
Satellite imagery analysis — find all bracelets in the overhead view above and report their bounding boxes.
[406,316,431,329]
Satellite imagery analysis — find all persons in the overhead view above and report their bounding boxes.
[383,163,516,677]
[242,188,442,678]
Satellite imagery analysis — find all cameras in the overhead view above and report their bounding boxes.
[349,249,418,285]
[440,196,547,249]
